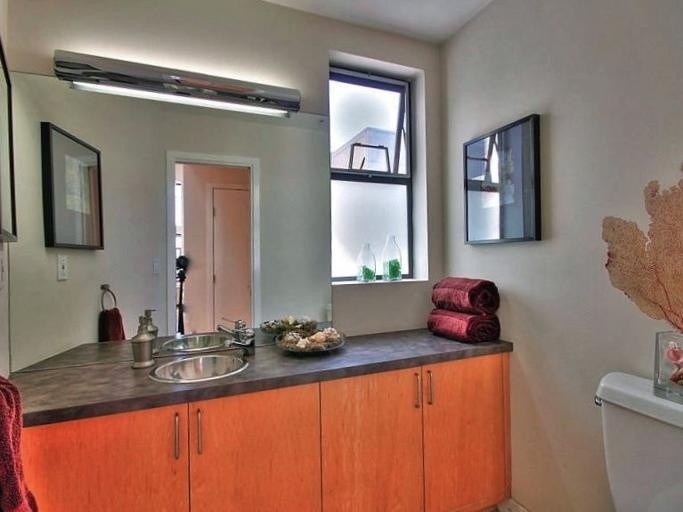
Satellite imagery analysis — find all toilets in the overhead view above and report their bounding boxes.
[594,372,683,512]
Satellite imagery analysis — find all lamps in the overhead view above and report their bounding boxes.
[50,49,304,121]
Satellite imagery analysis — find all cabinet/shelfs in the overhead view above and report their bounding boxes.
[320,352,513,511]
[21,380,321,512]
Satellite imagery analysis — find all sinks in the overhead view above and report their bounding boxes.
[148,355,248,384]
[163,333,227,351]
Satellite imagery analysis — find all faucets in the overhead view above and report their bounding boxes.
[225,329,255,355]
[216,317,246,337]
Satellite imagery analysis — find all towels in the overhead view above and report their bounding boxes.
[97,308,126,342]
[430,276,500,314]
[0,376,38,512]
[428,308,502,346]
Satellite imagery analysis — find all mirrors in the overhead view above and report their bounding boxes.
[5,69,333,379]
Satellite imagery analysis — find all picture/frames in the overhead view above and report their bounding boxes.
[458,111,543,246]
[0,39,18,244]
[37,120,105,251]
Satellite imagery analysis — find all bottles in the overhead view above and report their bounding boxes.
[381,234,402,282]
[355,241,376,282]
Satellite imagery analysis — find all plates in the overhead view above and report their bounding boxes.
[274,334,345,353]
[259,318,318,336]
